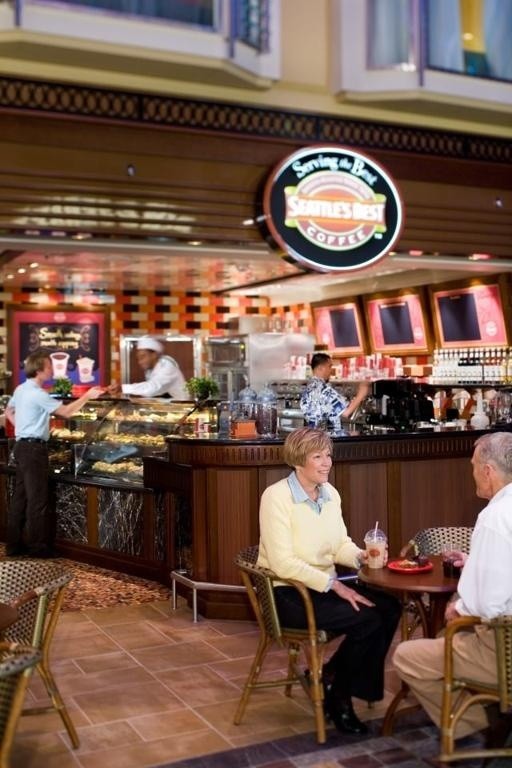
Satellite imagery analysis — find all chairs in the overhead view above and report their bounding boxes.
[0,556,81,768]
[232,542,374,744]
[436,615,512,767]
[398,524,476,702]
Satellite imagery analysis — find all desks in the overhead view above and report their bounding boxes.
[0,641,45,681]
[352,556,468,739]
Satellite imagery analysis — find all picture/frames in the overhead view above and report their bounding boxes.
[5,303,111,397]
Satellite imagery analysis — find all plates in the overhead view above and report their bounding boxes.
[388,558,434,573]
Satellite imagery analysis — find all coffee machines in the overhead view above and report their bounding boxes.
[366,379,434,427]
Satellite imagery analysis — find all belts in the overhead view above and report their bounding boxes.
[21,437,44,443]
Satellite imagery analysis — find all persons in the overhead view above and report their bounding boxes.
[4,350,108,557]
[105,334,188,400]
[390,431,511,767]
[254,426,402,733]
[297,352,370,428]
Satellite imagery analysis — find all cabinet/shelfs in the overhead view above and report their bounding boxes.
[1,399,219,480]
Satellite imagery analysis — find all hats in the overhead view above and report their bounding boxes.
[137,337,162,353]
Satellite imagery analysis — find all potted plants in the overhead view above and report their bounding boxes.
[184,376,220,404]
[53,376,74,397]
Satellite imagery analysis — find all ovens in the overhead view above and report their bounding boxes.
[206,332,317,401]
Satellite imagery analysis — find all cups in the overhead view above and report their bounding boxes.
[364,528,388,568]
[441,540,462,578]
[50,352,70,380]
[76,359,95,382]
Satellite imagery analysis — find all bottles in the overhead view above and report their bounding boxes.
[238,380,258,421]
[432,346,511,386]
[255,380,279,437]
[218,404,231,437]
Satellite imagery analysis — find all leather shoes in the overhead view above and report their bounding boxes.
[325,697,372,739]
[305,666,330,721]
